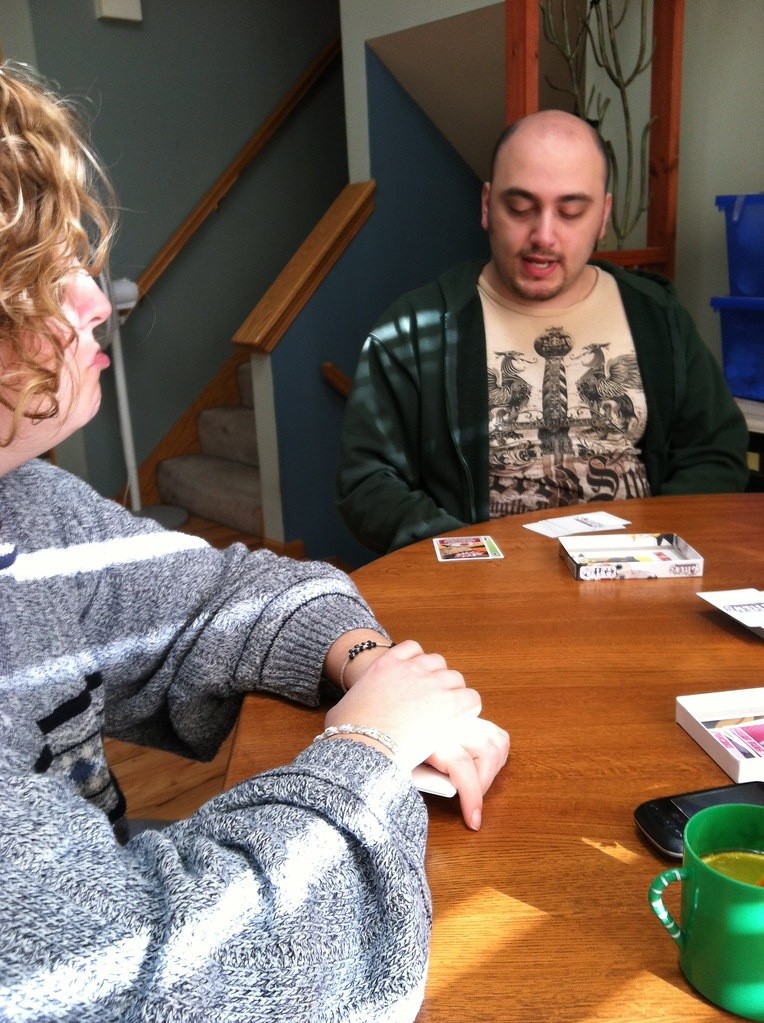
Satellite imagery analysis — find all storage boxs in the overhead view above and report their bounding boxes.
[708,194,764,493]
[558,532,705,580]
[676,687,764,784]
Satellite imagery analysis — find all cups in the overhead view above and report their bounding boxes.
[648,803,764,1023]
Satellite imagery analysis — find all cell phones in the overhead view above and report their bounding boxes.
[632,779,764,860]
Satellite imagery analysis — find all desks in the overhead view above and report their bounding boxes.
[235,493,764,1023]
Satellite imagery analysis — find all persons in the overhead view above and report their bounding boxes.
[0,64,510,1023]
[336,109,747,560]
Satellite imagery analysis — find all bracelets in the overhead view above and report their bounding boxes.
[338,641,397,693]
[313,724,400,760]
